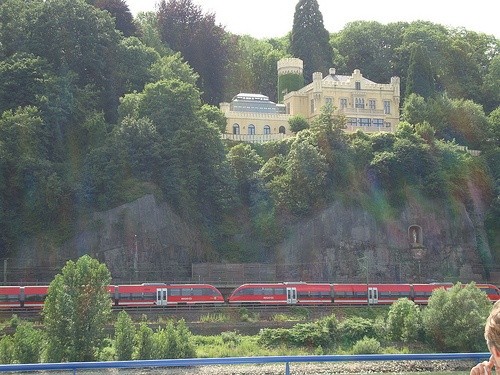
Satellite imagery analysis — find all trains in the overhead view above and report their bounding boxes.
[0,285,500,309]
